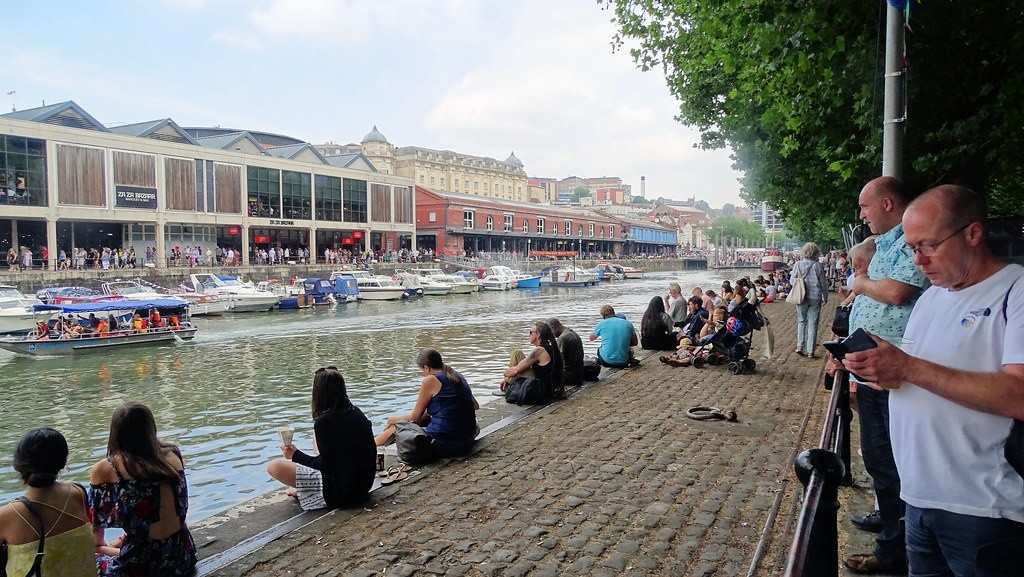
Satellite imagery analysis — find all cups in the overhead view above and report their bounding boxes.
[875,334,915,389]
[277,427,295,448]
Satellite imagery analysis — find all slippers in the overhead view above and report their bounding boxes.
[378,463,413,477]
[381,467,408,485]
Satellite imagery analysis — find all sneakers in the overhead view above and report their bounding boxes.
[850,511,884,533]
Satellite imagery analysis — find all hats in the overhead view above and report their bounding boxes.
[677,337,695,349]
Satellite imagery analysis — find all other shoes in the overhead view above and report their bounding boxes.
[668,359,688,368]
[843,550,906,573]
[795,347,803,354]
[659,355,668,363]
[807,353,815,358]
[629,356,640,363]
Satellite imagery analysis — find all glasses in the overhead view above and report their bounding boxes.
[530,330,543,335]
[901,221,978,257]
[314,366,337,376]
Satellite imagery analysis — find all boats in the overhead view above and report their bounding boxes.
[182,263,646,313]
[0,277,233,357]
[761,248,788,271]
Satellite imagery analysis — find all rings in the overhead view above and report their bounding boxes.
[827,367,831,370]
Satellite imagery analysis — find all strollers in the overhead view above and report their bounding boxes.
[689,283,767,375]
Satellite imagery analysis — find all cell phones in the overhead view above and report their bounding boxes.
[823,328,878,381]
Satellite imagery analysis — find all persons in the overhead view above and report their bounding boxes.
[88,402,197,577]
[374,349,476,465]
[0,427,98,577]
[589,304,638,368]
[826,176,931,575]
[266,366,377,511]
[254,246,710,265]
[7,246,33,272]
[819,249,850,293]
[492,318,584,405]
[641,268,792,366]
[0,187,31,202]
[56,309,181,341]
[31,320,50,340]
[713,250,794,267]
[790,242,829,358]
[40,245,240,271]
[843,184,1024,577]
[248,199,320,220]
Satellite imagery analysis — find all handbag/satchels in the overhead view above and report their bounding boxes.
[505,377,540,406]
[831,305,852,338]
[756,301,774,359]
[786,276,806,305]
[392,419,432,465]
